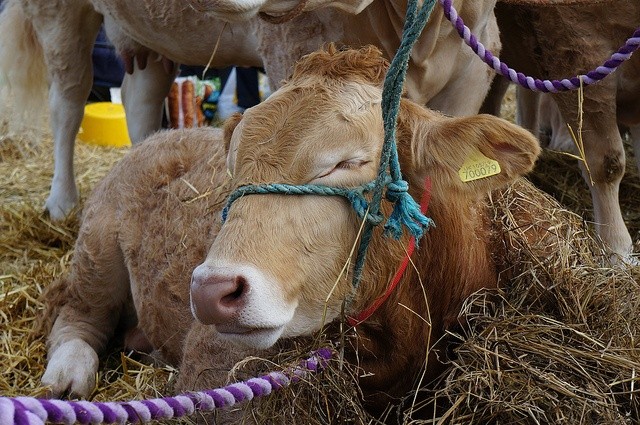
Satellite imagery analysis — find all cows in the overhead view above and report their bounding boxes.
[478,0,640,272]
[35,41,542,425]
[0,1,501,228]
